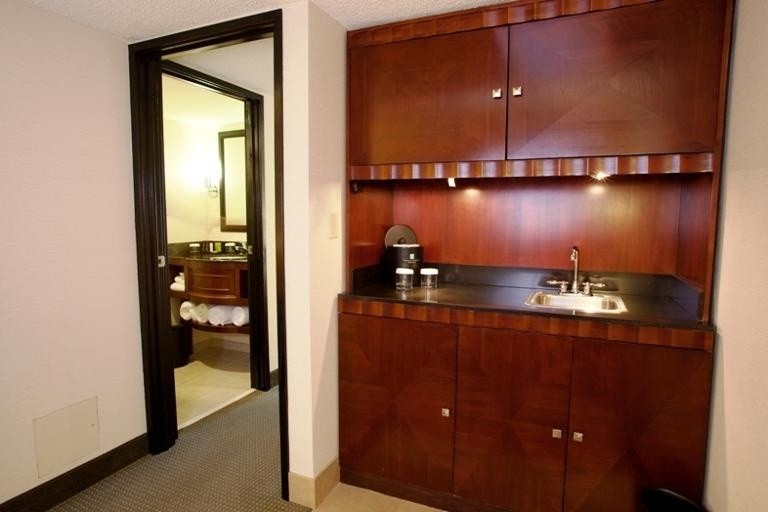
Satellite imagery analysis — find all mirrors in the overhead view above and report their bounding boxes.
[218,129,247,232]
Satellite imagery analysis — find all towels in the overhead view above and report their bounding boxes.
[179,300,250,328]
[169,272,185,292]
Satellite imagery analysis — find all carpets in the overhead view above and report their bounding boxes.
[45,384,314,511]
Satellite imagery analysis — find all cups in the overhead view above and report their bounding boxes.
[420,268,439,290]
[396,268,414,291]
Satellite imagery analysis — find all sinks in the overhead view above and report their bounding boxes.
[525,289,630,314]
[212,255,247,261]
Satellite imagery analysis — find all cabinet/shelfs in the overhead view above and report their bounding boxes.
[339,311,456,512]
[347,1,729,166]
[453,323,713,511]
[168,264,250,354]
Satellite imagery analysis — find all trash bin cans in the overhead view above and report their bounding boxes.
[635,487,707,512]
[169,325,189,368]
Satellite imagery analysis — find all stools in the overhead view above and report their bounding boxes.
[168,325,194,368]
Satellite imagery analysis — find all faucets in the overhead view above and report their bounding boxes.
[236,245,248,254]
[570,244,581,294]
